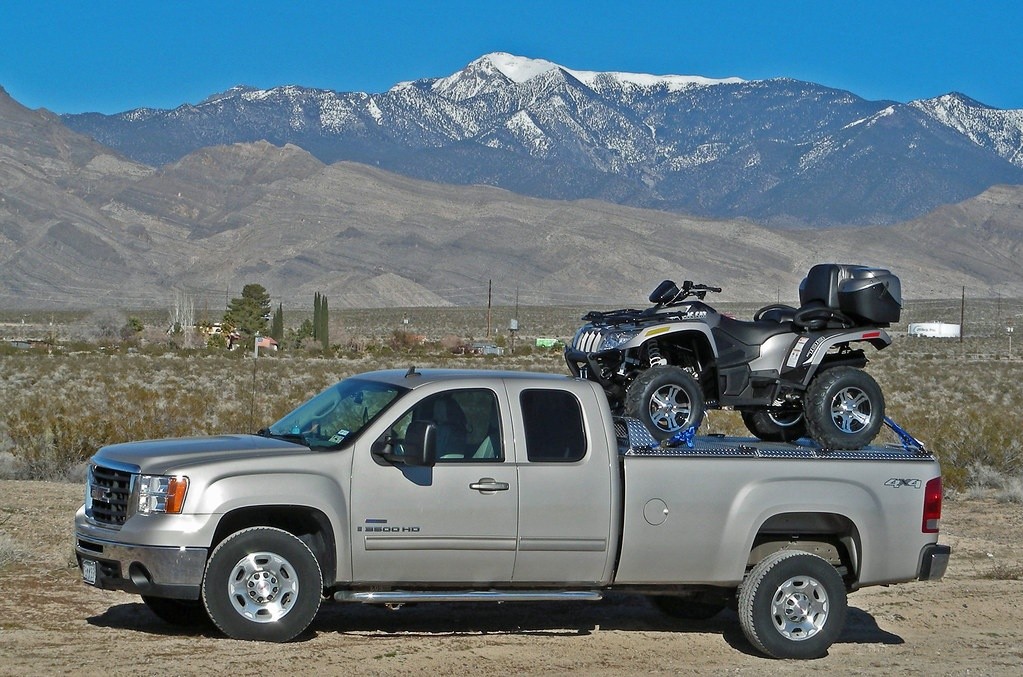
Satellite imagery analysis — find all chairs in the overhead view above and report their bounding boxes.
[426,397,467,455]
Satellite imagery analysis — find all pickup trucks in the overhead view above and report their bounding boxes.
[73,363,951,659]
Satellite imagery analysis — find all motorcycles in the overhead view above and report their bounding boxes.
[563,263,902,451]
